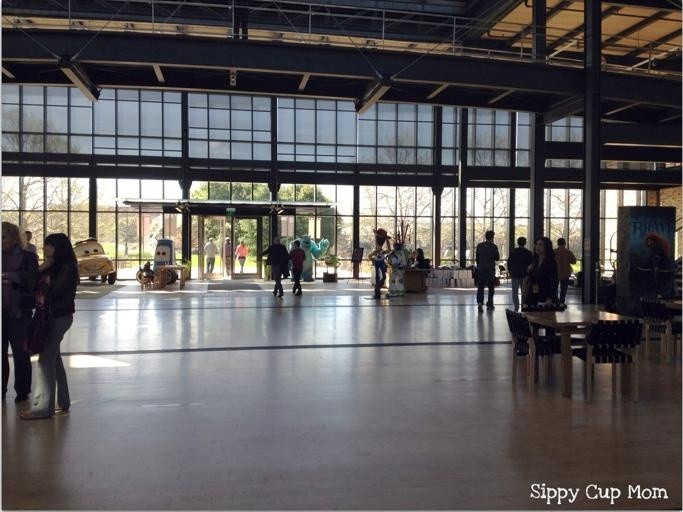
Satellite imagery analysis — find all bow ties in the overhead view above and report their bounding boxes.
[54,406,70,415]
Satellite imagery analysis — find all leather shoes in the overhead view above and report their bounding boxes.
[15,394,30,403]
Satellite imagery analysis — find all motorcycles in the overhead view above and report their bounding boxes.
[135,261,175,286]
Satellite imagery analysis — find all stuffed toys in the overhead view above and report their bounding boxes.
[368,228,391,299]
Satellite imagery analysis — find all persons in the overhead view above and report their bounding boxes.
[235,240,249,273]
[204,237,219,272]
[21,231,38,252]
[413,247,430,284]
[288,240,306,296]
[256,238,290,297]
[476,231,499,312]
[645,230,670,272]
[220,238,236,275]
[2,222,38,402]
[19,233,81,420]
[515,237,558,386]
[507,237,532,312]
[552,237,576,302]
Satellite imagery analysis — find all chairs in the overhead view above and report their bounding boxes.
[140,267,154,291]
[505,294,681,405]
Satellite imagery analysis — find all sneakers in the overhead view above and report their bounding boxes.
[18,411,52,420]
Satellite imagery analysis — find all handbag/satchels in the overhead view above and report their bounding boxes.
[22,303,51,357]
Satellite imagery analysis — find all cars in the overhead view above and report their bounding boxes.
[72,237,115,286]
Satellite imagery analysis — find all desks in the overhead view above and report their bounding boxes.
[157,264,188,290]
[403,268,428,294]
